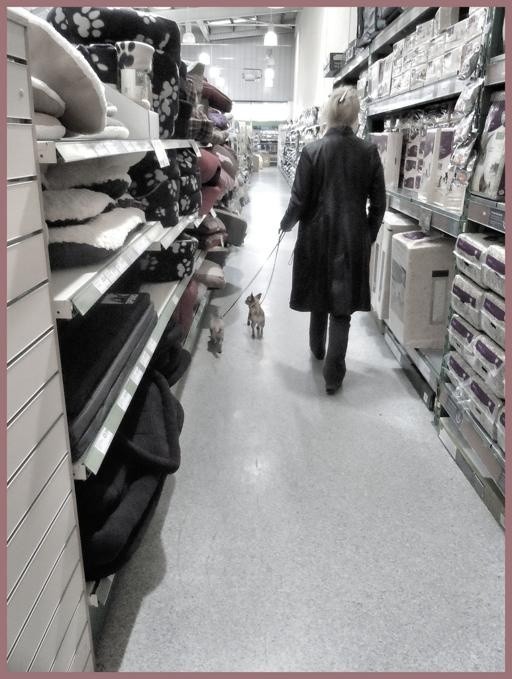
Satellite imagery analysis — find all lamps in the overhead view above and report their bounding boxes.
[264,6,280,87]
[182,23,212,68]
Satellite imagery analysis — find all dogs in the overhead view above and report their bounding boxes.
[245,292,265,339]
[205,303,224,353]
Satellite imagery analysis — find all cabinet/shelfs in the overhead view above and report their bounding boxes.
[28,102,233,620]
[277,4,507,534]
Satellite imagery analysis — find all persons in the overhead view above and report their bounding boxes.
[280,85,386,396]
